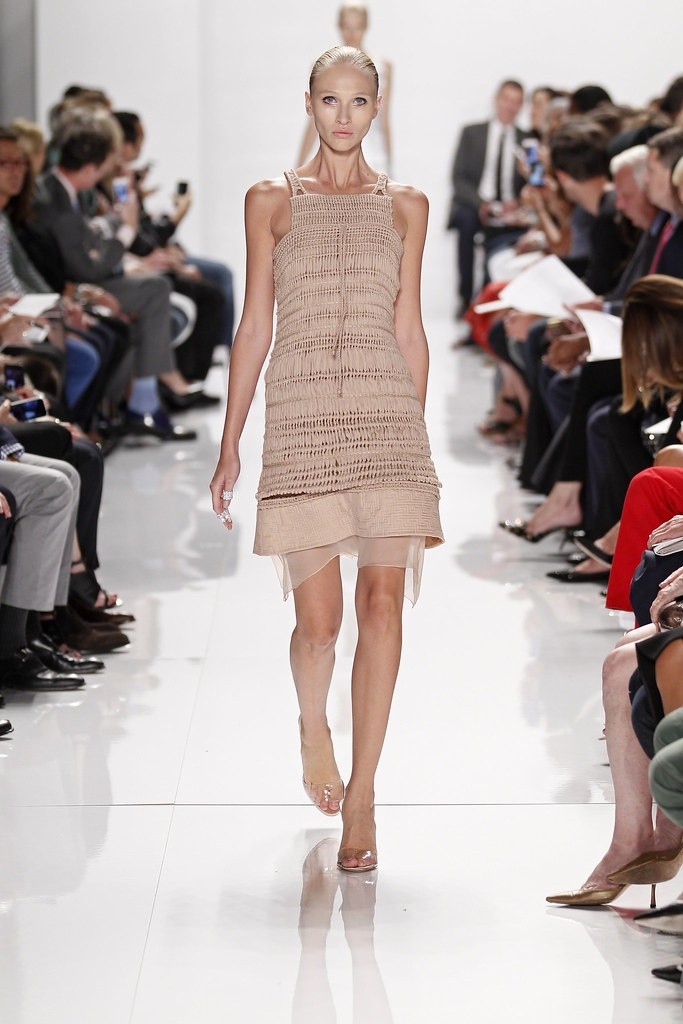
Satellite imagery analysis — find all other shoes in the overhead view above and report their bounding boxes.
[546,568,610,585]
[40,617,130,651]
[574,538,613,567]
[68,589,135,624]
[453,331,525,469]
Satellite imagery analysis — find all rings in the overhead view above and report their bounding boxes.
[217,508,232,523]
[221,491,233,500]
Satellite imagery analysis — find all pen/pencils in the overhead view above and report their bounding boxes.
[12,313,44,329]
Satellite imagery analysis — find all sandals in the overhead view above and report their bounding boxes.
[70,560,116,609]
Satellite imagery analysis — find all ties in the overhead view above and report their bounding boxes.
[495,135,502,200]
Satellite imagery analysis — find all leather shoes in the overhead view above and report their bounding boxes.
[0,649,84,691]
[200,396,219,407]
[0,720,14,736]
[128,417,197,441]
[157,380,203,410]
[29,639,105,674]
[635,904,683,934]
[652,964,683,984]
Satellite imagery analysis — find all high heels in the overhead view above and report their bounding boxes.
[499,519,580,551]
[606,844,683,884]
[338,799,378,871]
[546,885,656,908]
[300,726,343,815]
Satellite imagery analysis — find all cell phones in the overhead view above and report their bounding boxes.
[9,397,48,422]
[178,182,187,195]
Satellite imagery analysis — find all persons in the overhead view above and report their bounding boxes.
[0,85,236,737]
[296,0,393,173]
[208,47,435,874]
[454,78,683,984]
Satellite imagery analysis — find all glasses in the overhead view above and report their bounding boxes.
[0,157,27,168]
